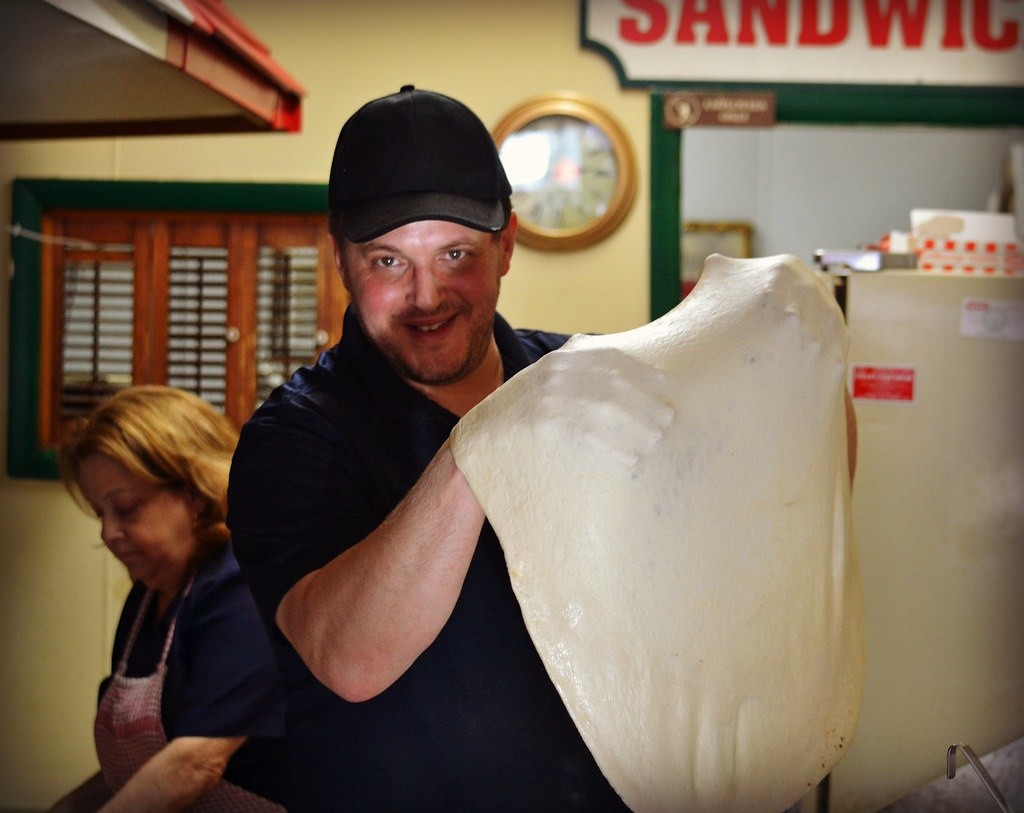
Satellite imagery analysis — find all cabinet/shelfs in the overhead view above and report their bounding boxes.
[827,272,1024,813]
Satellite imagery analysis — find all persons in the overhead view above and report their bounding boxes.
[46,384,299,813]
[226,86,857,813]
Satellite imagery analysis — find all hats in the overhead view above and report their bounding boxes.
[327,85,512,243]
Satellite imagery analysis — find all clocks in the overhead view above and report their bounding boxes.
[494,96,636,253]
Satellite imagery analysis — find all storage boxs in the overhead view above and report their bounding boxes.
[908,208,1024,277]
